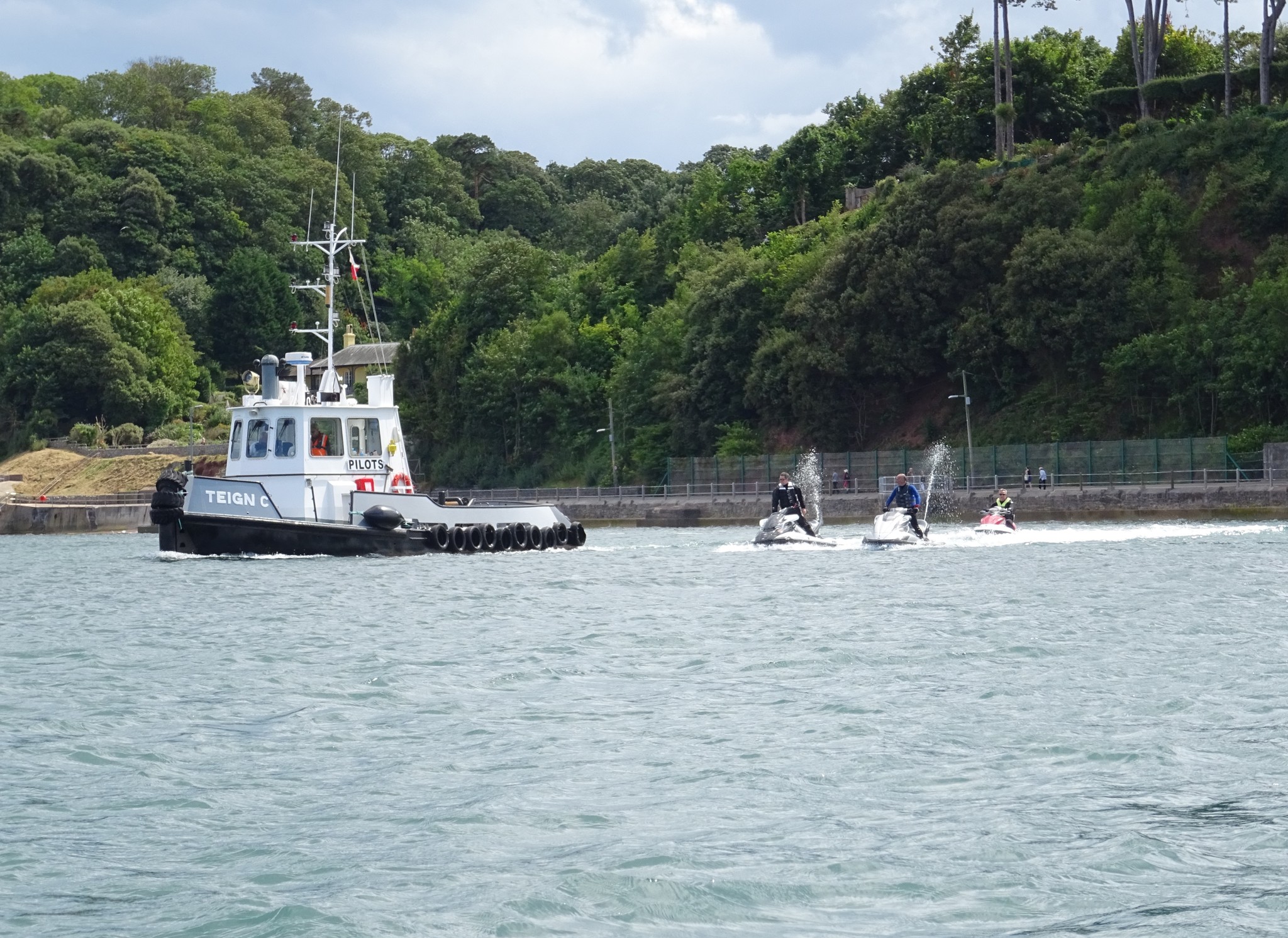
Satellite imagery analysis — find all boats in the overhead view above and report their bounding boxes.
[137,176,587,557]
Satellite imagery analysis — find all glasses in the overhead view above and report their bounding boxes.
[999,493,1005,496]
[779,479,785,481]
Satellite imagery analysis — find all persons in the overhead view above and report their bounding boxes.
[843,469,850,494]
[254,431,268,457]
[310,423,331,456]
[982,488,1015,531]
[1038,467,1047,490]
[1023,466,1032,490]
[919,470,926,491]
[831,469,840,495]
[907,468,917,491]
[771,472,816,537]
[883,474,924,539]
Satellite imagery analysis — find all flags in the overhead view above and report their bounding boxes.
[349,253,360,280]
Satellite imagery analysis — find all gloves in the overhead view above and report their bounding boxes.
[883,508,888,513]
[912,509,918,514]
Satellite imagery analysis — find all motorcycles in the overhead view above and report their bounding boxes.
[973,505,1017,534]
[750,502,824,544]
[861,506,932,546]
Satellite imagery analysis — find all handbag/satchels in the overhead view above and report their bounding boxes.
[846,473,850,481]
[1024,474,1029,481]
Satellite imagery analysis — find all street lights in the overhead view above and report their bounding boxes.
[596,428,618,495]
[948,394,975,487]
[189,405,204,470]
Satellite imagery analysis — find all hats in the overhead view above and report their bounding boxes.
[844,469,848,473]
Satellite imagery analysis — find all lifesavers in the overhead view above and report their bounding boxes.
[449,525,467,553]
[430,524,450,551]
[391,472,414,494]
[479,522,497,551]
[552,522,568,546]
[148,507,185,524]
[507,521,528,550]
[465,525,483,551]
[155,468,189,496]
[496,525,513,552]
[571,521,586,546]
[541,526,557,549]
[150,490,185,508]
[527,524,543,550]
